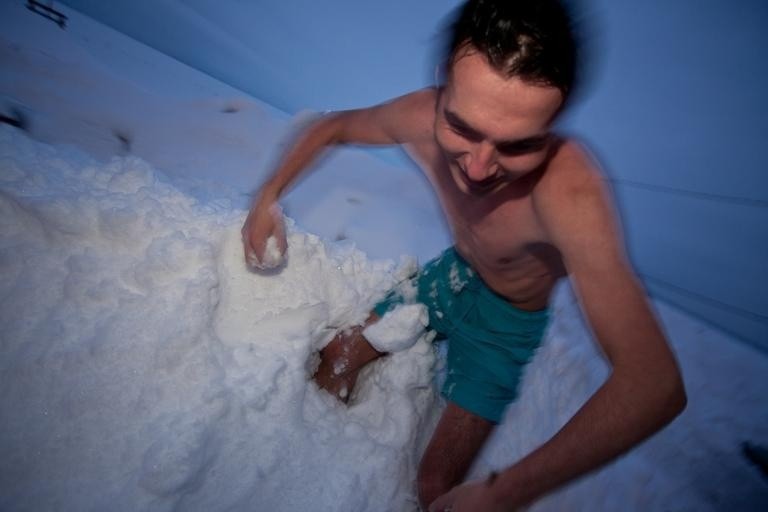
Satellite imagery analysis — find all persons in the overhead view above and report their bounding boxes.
[242,0,687,512]
